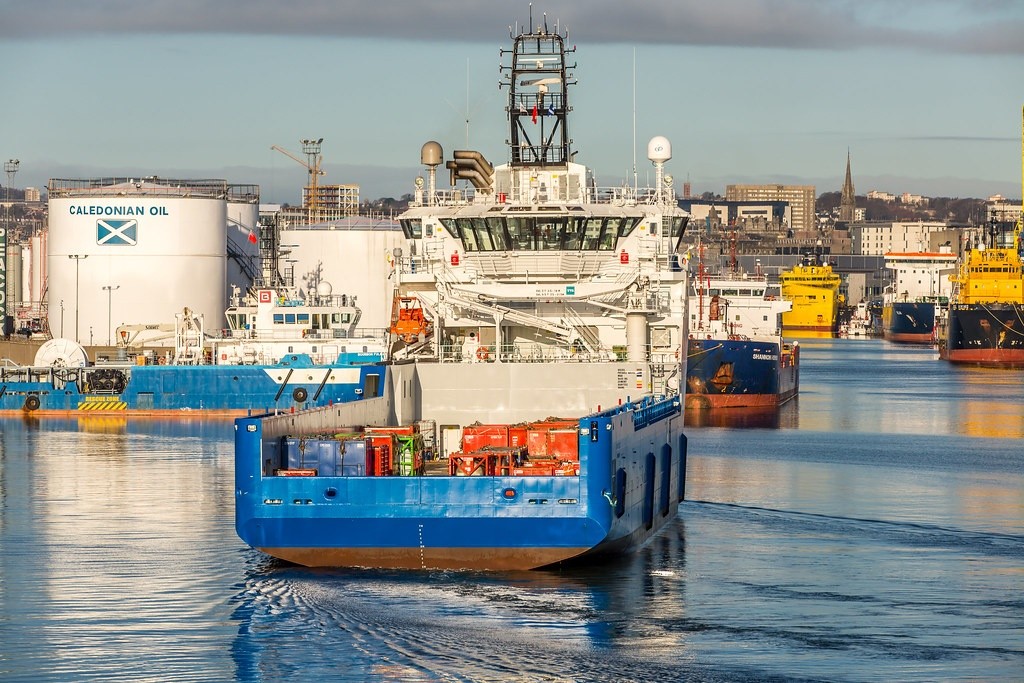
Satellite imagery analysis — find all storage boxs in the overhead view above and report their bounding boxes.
[398,435,424,477]
[462,424,508,451]
[281,437,318,469]
[362,425,414,436]
[273,469,317,477]
[514,464,553,476]
[527,418,579,462]
[478,447,527,466]
[318,439,372,477]
[372,445,390,476]
[553,467,579,476]
[334,433,399,474]
[509,427,527,447]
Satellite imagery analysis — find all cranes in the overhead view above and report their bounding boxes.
[270,144,326,213]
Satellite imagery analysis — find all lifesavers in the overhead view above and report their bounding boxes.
[292,388,308,403]
[25,395,40,411]
[157,357,166,366]
[86,370,124,391]
[475,346,489,360]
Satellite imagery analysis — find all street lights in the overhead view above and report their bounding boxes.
[67,254,89,342]
[101,286,120,346]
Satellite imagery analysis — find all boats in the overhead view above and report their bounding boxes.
[0,213,386,415]
[883,297,934,344]
[937,217,1024,362]
[234,3,693,573]
[684,217,800,407]
[779,252,841,331]
